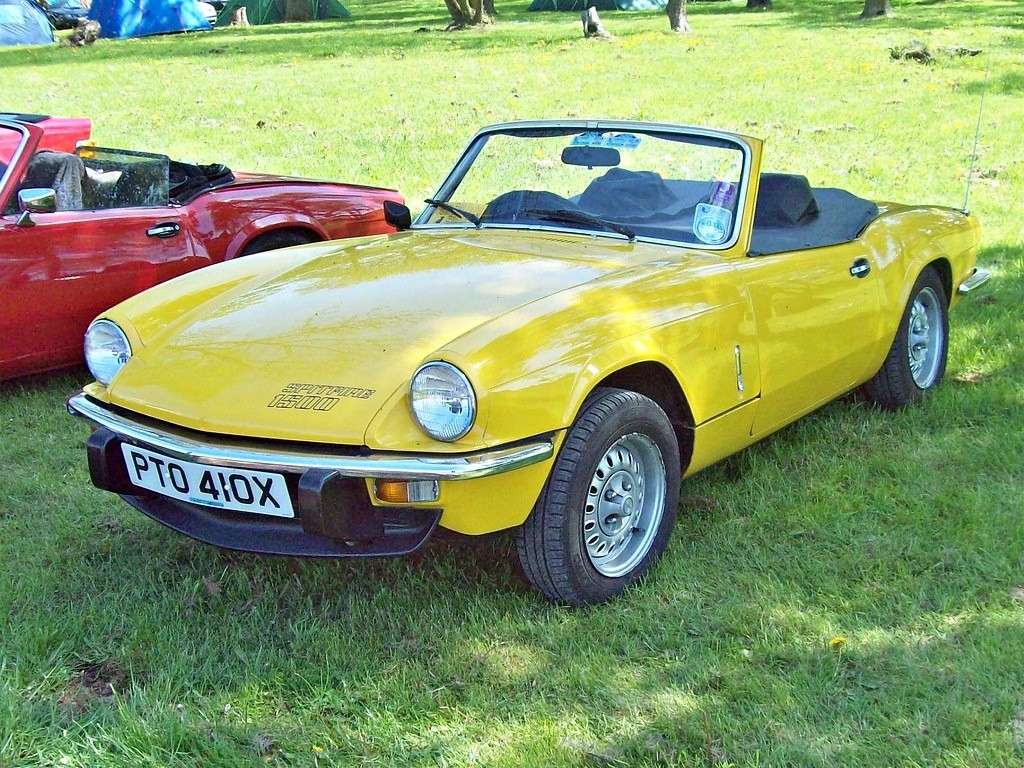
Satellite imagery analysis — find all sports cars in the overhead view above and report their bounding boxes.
[70,118,992,607]
[0,109,407,390]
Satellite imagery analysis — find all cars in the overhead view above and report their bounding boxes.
[0,113,96,182]
